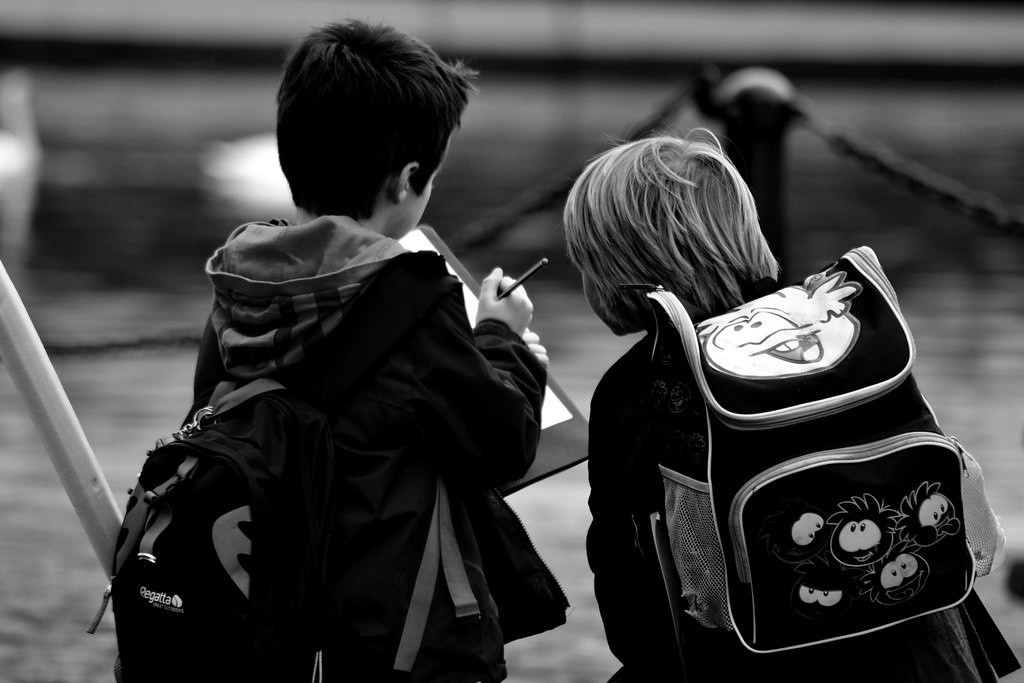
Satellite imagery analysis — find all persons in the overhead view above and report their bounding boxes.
[566,138,1021,683]
[181,20,574,683]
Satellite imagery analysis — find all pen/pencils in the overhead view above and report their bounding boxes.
[498,257,549,300]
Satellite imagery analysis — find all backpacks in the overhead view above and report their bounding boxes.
[646,245,1003,664]
[89,270,460,683]
